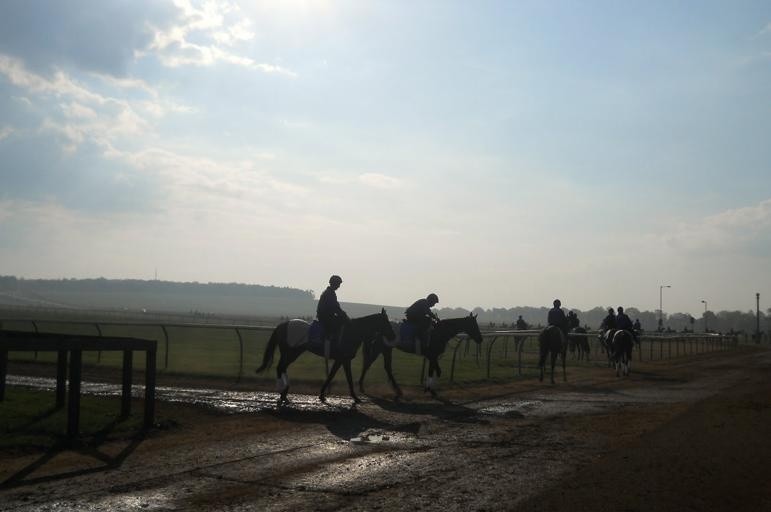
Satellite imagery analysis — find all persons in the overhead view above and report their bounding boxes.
[406,294,439,343]
[517,316,527,329]
[317,275,347,360]
[548,300,568,352]
[567,307,640,359]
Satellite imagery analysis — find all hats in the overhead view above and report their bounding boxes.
[427,294,439,304]
[328,275,343,283]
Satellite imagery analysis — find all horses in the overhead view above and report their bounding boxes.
[536,311,580,384]
[254,307,396,405]
[611,330,634,377]
[358,312,483,399]
[568,328,591,363]
[603,329,621,369]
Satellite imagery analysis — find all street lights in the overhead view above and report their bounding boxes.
[660,284,671,333]
[702,299,708,333]
[755,291,760,338]
